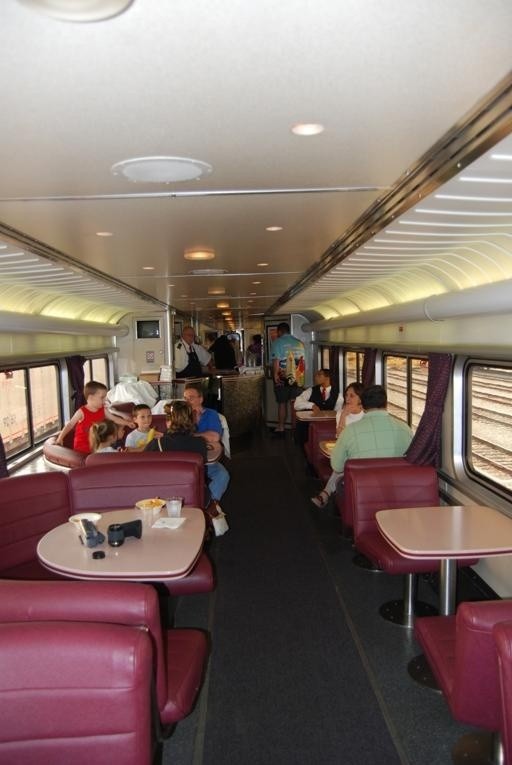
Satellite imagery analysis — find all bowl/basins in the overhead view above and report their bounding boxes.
[68,512,103,530]
[135,498,165,515]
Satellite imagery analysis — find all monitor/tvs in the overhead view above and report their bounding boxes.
[135,320,159,338]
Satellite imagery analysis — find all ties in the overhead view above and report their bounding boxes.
[322,387,327,400]
[189,345,193,353]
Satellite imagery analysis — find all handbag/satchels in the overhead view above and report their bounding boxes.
[208,503,229,537]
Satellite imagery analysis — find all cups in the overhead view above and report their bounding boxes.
[165,497,183,518]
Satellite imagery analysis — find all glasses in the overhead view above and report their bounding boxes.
[185,395,200,401]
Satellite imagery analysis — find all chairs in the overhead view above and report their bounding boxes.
[306,423,335,479]
[348,468,482,627]
[336,457,428,536]
[416,598,512,728]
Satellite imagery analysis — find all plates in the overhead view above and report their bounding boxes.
[326,443,338,450]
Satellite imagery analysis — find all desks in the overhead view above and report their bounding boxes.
[298,409,340,422]
[377,505,511,691]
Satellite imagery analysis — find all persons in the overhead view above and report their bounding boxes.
[182,382,230,505]
[126,404,161,451]
[246,334,264,361]
[310,382,367,509]
[331,386,415,476]
[205,331,237,370]
[52,381,136,454]
[110,414,125,451]
[270,323,309,439]
[90,420,120,452]
[173,327,213,377]
[142,400,228,535]
[293,367,345,412]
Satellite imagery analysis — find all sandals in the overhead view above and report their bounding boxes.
[292,429,298,435]
[271,429,284,435]
[311,489,330,509]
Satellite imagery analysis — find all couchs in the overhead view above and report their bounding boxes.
[0,467,218,593]
[0,581,209,731]
[0,621,156,765]
[44,414,206,474]
